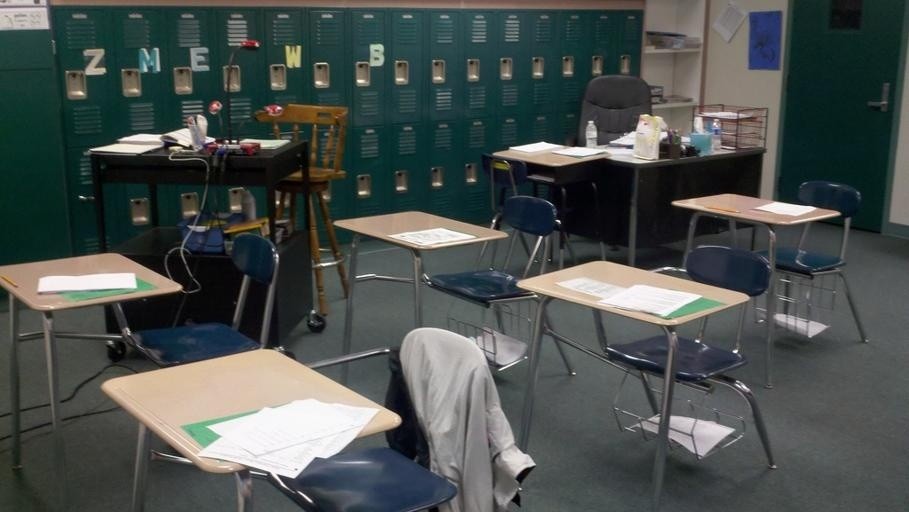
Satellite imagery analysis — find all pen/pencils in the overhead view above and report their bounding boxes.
[667,128,681,145]
[188,115,204,146]
[0,274,19,287]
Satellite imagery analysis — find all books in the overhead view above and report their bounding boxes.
[117,129,216,149]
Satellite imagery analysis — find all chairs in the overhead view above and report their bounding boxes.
[607,244,778,470]
[430,196,577,377]
[254,104,348,316]
[577,74,652,148]
[268,326,488,511]
[129,234,280,367]
[751,180,871,344]
[481,154,579,268]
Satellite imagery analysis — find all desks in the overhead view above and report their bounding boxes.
[481,138,767,266]
[1,253,184,511]
[670,193,842,388]
[516,260,751,511]
[331,210,509,354]
[99,348,402,512]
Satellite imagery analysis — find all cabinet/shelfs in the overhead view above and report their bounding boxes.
[345,7,500,222]
[88,141,326,360]
[640,2,708,133]
[589,10,642,80]
[497,9,590,155]
[47,1,349,260]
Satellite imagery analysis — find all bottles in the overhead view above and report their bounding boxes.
[243,190,256,221]
[583,120,599,149]
[711,119,722,149]
[671,129,681,144]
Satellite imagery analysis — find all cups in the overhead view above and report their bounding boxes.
[188,123,207,148]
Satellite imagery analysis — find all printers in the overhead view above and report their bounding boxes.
[176,211,244,253]
